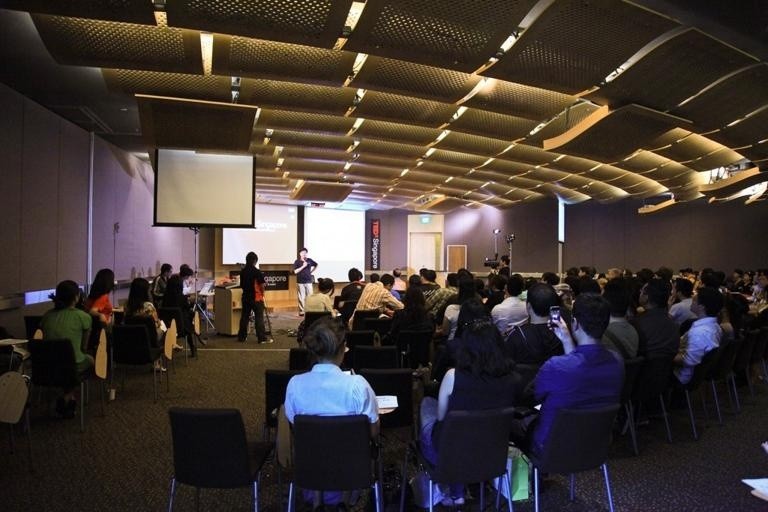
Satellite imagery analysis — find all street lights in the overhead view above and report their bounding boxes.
[412,465,447,509]
[301,489,363,508]
[494,445,530,500]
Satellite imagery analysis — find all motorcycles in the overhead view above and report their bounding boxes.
[245,301,275,338]
[192,230,216,333]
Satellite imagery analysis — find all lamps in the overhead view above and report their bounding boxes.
[166,406,278,512]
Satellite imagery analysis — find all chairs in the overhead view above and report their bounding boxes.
[0,304,115,475]
[166,406,278,512]
[400,407,513,512]
[287,415,384,512]
[259,339,273,344]
[107,278,212,403]
[265,287,766,457]
[495,403,621,512]
[445,494,465,505]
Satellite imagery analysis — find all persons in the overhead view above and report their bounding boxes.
[0,262,200,431]
[292,248,319,316]
[496,254,512,277]
[237,252,274,344]
[286,267,768,510]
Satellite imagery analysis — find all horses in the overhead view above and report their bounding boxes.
[550,306,560,327]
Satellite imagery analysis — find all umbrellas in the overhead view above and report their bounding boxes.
[152,367,167,373]
[173,344,184,352]
